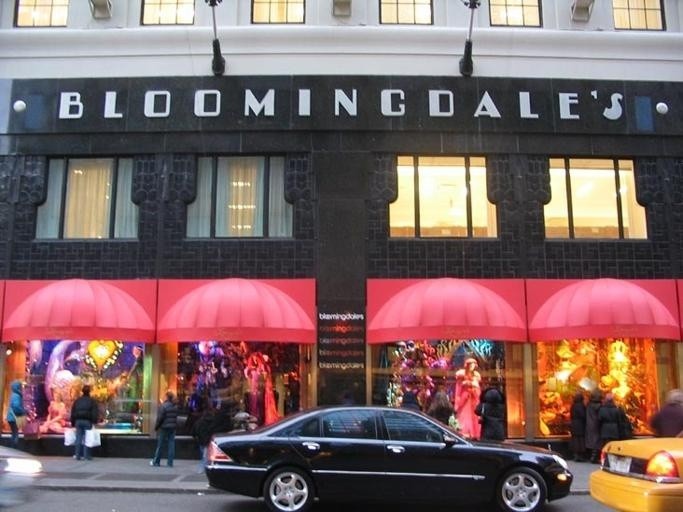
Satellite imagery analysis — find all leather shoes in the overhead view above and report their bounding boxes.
[590,455,600,464]
[574,455,585,462]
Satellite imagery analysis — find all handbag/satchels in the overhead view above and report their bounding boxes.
[64,427,77,447]
[84,426,102,447]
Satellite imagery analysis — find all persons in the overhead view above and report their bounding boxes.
[586,392,602,464]
[570,393,587,462]
[598,393,620,446]
[150,392,178,467]
[650,389,683,437]
[455,359,481,441]
[427,392,453,425]
[474,387,505,441]
[401,392,422,412]
[6,381,27,450]
[616,407,633,440]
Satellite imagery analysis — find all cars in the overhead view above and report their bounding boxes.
[590,431,683,512]
[204,406,573,512]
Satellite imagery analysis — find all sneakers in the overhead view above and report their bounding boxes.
[165,463,172,468]
[149,460,158,466]
[83,456,91,462]
[72,454,80,460]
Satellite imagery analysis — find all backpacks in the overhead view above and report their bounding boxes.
[8,404,27,430]
[70,385,98,461]
